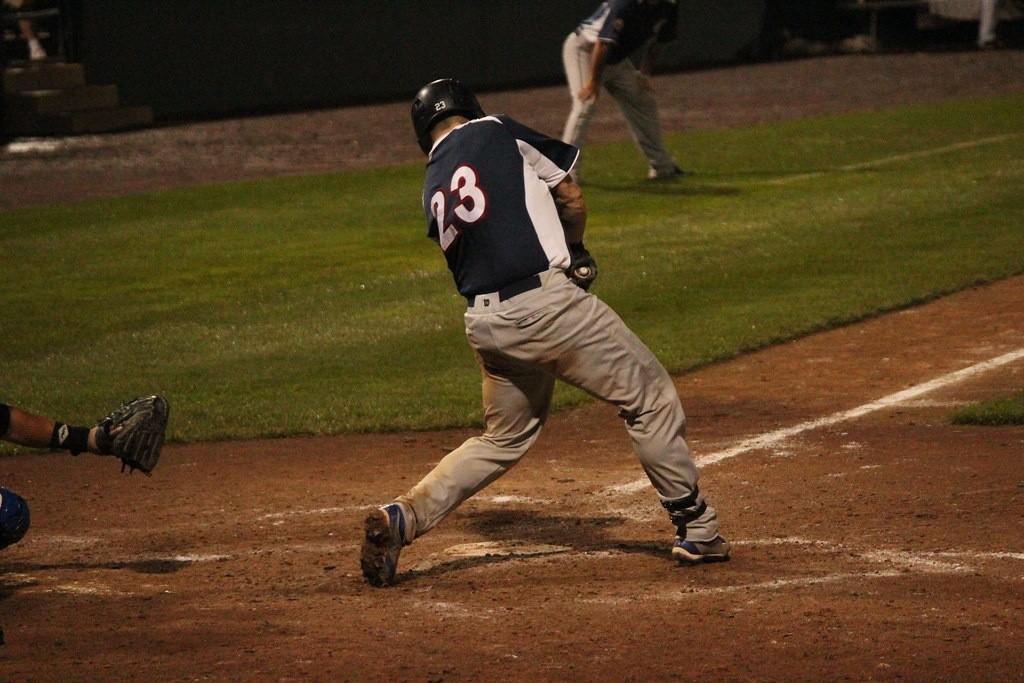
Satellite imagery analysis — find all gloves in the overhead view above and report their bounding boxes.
[568,241,598,290]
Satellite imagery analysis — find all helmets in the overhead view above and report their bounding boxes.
[410,78,486,155]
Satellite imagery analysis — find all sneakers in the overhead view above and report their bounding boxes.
[360,505,404,586]
[671,534,730,564]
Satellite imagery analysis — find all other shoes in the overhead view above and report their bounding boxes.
[28,38,45,61]
[649,160,684,180]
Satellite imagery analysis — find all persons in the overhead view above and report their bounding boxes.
[354,77,731,587]
[5,0,47,61]
[561,0,703,181]
[927,0,1000,51]
[0,391,168,549]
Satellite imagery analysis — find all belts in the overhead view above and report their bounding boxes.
[466,275,542,307]
[574,26,581,35]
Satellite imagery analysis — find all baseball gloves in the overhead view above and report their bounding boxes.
[97,393,170,476]
